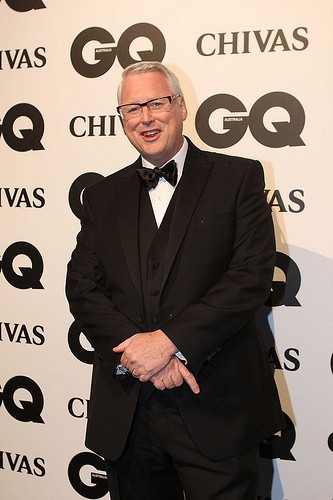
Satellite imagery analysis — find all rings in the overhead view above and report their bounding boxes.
[133,371,138,376]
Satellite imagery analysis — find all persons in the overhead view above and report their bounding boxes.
[66,61,286,499]
